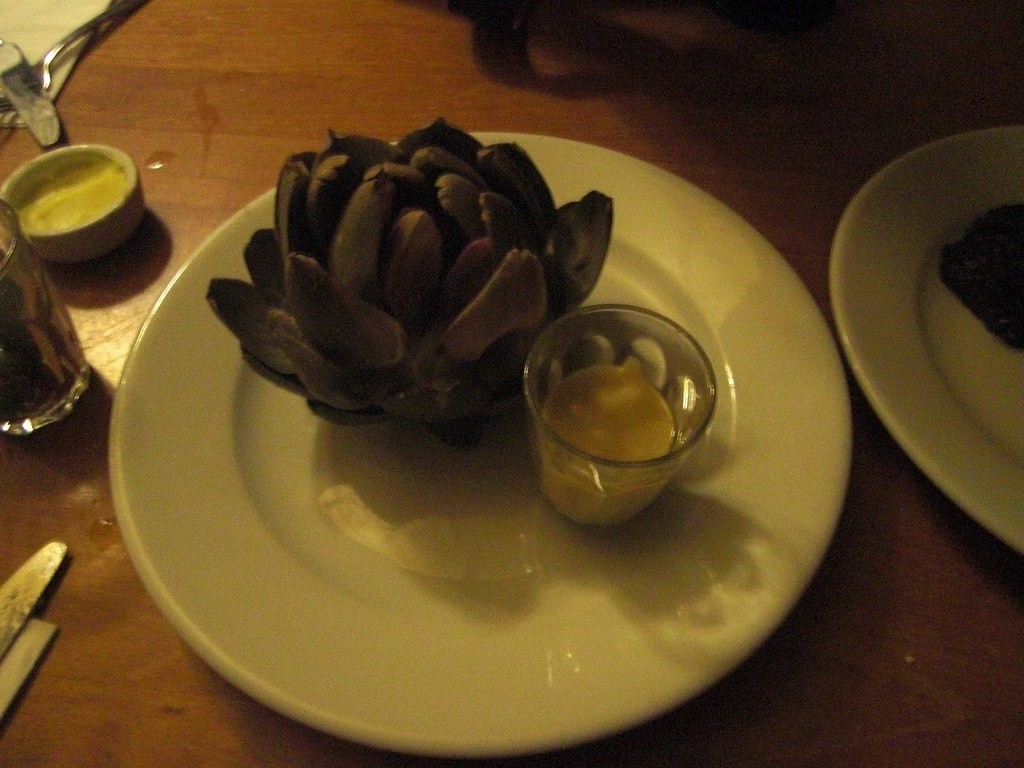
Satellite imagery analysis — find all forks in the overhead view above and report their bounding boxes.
[0,0,151,114]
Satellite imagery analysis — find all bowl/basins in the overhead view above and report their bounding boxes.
[1,143,145,261]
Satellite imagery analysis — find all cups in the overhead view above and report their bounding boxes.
[524,304,719,527]
[0,204,95,437]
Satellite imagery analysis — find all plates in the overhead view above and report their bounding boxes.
[831,125,1024,559]
[107,133,854,758]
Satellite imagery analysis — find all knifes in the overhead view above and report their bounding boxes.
[0,542,68,658]
[0,37,60,147]
